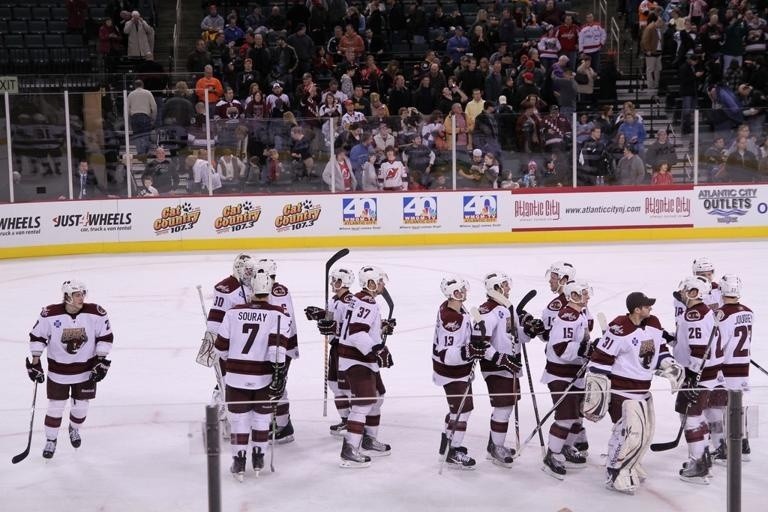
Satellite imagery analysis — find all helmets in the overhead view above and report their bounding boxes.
[256,259,277,278]
[328,268,355,290]
[359,265,389,293]
[564,280,593,303]
[232,253,254,285]
[484,271,513,299]
[720,274,744,298]
[693,257,714,277]
[673,276,711,305]
[251,273,272,296]
[543,260,575,293]
[440,276,470,301]
[61,279,88,306]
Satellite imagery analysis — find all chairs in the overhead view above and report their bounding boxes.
[29,21,48,34]
[204,0,222,8]
[288,0,305,6]
[69,48,89,63]
[46,35,64,48]
[246,0,264,7]
[11,21,28,34]
[13,8,32,20]
[408,28,425,51]
[86,0,97,8]
[98,0,108,8]
[0,35,5,48]
[6,35,23,48]
[481,4,496,14]
[226,7,242,17]
[39,0,57,7]
[66,34,83,48]
[556,2,571,10]
[0,49,9,65]
[268,7,284,16]
[226,0,242,8]
[531,3,540,10]
[359,61,379,71]
[17,0,37,8]
[404,0,416,3]
[89,36,95,46]
[33,8,51,20]
[443,4,458,14]
[388,30,410,53]
[318,78,336,86]
[424,4,439,13]
[464,16,477,30]
[30,49,49,65]
[49,48,67,64]
[25,34,44,49]
[424,0,437,4]
[268,0,284,6]
[492,43,510,51]
[246,8,265,16]
[58,0,68,7]
[428,29,448,50]
[382,61,402,71]
[90,9,105,16]
[0,20,10,34]
[442,0,456,4]
[404,3,417,13]
[10,49,29,66]
[462,4,480,15]
[139,0,154,11]
[404,61,426,71]
[513,43,526,51]
[52,8,70,21]
[49,21,67,34]
[0,0,16,8]
[141,9,157,32]
[0,9,12,19]
[294,79,317,86]
[205,9,223,16]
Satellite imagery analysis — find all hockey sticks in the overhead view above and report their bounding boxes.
[197,283,226,419]
[515,289,547,458]
[323,248,350,417]
[437,307,486,474]
[11,379,39,465]
[269,315,281,473]
[650,311,723,452]
[380,286,394,350]
[511,312,609,459]
[486,288,520,452]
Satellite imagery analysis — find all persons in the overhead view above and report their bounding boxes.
[312,45,333,69]
[296,68,393,121]
[578,127,614,185]
[690,258,725,457]
[260,147,273,183]
[637,0,663,29]
[321,147,358,193]
[473,101,504,180]
[401,134,436,185]
[247,34,271,73]
[397,118,421,136]
[430,174,449,190]
[414,0,547,102]
[244,83,268,107]
[126,80,158,134]
[500,168,520,189]
[408,171,428,191]
[57,160,121,201]
[142,146,181,195]
[350,131,377,190]
[538,103,573,153]
[575,54,599,105]
[426,124,448,153]
[377,145,409,192]
[761,135,768,177]
[253,258,300,446]
[520,94,543,118]
[518,262,605,459]
[304,268,356,437]
[247,89,268,118]
[215,271,294,481]
[172,80,196,103]
[443,102,475,150]
[514,102,541,154]
[266,35,298,79]
[184,155,223,197]
[651,161,674,185]
[136,174,160,198]
[186,148,209,196]
[557,13,579,76]
[289,24,316,66]
[334,122,364,155]
[539,158,563,187]
[187,100,218,158]
[186,38,212,73]
[464,90,487,149]
[361,152,382,192]
[386,71,413,114]
[626,134,643,158]
[582,292,684,494]
[578,12,607,71]
[338,265,396,468]
[551,53,571,78]
[639,12,665,88]
[387,58,403,69]
[221,107,248,164]
[269,149,292,182]
[236,58,262,96]
[469,272,544,470]
[214,85,244,119]
[642,275,725,486]
[186,2,414,31]
[282,110,298,136]
[423,109,441,143]
[706,135,726,181]
[326,24,344,56]
[99,17,123,56]
[540,22,561,71]
[196,64,223,118]
[617,112,647,145]
[26,279,115,458]
[285,125,317,177]
[160,86,196,154]
[641,128,679,170]
[216,148,246,184]
[271,99,289,118]
[711,275,754,460]
[339,23,367,56]
[361,25,387,58]
[725,136,760,186]
[494,94,514,114]
[542,280,615,481]
[614,143,646,186]
[362,56,383,78]
[338,49,358,69]
[247,154,261,184]
[711,148,730,181]
[110,153,139,198]
[267,81,293,118]
[520,160,540,188]
[205,254,255,441]
[734,123,758,157]
[457,147,485,186]
[1,171,30,204]
[372,122,396,151]
[431,274,491,471]
[270,135,287,150]
[124,11,154,62]
[666,0,768,124]
[479,153,500,188]
[607,130,628,177]
[399,106,427,133]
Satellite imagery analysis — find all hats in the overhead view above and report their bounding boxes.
[499,95,506,105]
[626,292,657,312]
[472,148,483,157]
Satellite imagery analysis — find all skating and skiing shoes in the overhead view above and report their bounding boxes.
[679,468,709,485]
[43,439,56,464]
[268,422,295,445]
[486,441,517,460]
[330,418,348,436]
[605,477,638,496]
[490,445,514,468]
[359,436,391,456]
[710,441,729,468]
[457,446,472,456]
[682,459,714,477]
[230,452,247,484]
[68,424,82,452]
[339,442,371,469]
[742,438,751,462]
[252,447,264,478]
[445,448,476,470]
[563,447,588,469]
[541,448,567,480]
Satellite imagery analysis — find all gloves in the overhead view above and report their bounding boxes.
[579,339,601,361]
[89,357,111,382]
[519,311,533,327]
[461,341,491,362]
[381,318,396,336]
[374,344,394,369]
[580,375,611,422]
[26,357,45,383]
[491,352,522,375]
[523,319,548,339]
[317,319,338,336]
[265,363,287,400]
[680,382,699,405]
[658,359,685,394]
[304,306,326,321]
